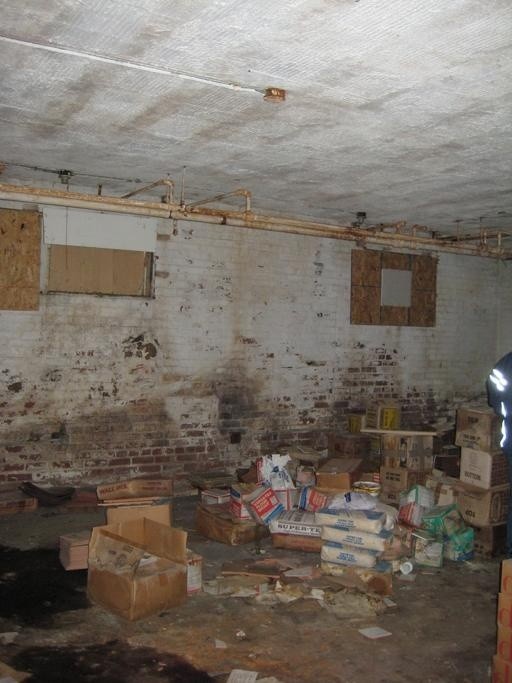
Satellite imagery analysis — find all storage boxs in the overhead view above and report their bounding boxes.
[88,518,187,620]
[455,406,502,451]
[366,405,400,429]
[460,447,507,487]
[383,452,434,470]
[458,485,508,526]
[473,525,508,558]
[379,466,426,490]
[315,458,363,490]
[104,505,170,526]
[381,434,435,454]
